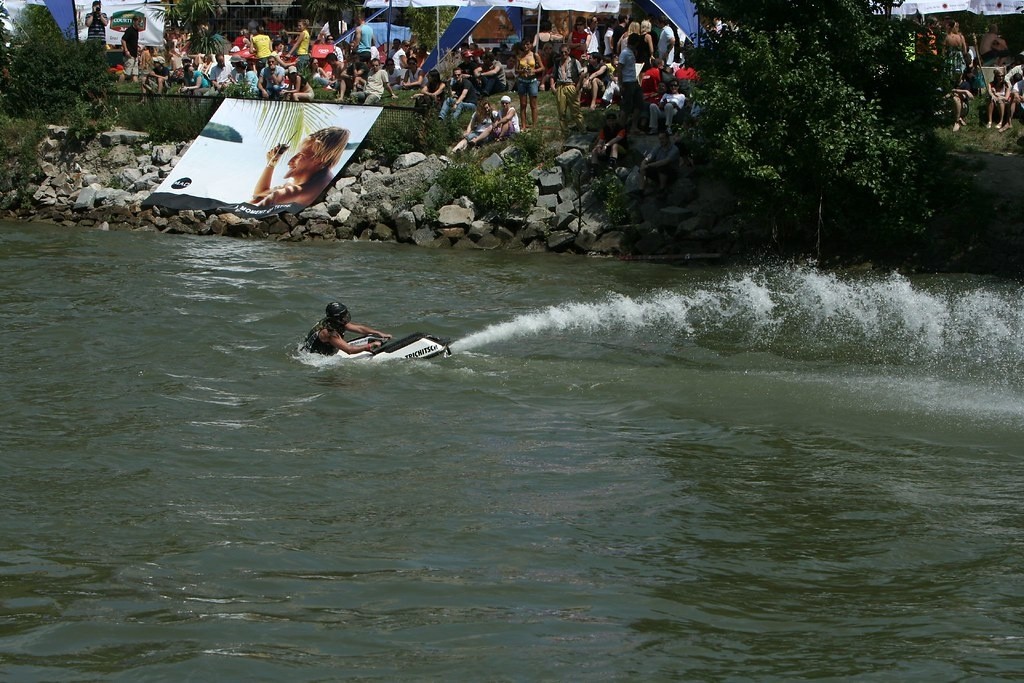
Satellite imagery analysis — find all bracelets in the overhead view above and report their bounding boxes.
[392,94,394,96]
[368,344,371,350]
[456,103,459,105]
[588,77,591,81]
[480,72,481,75]
[604,144,608,147]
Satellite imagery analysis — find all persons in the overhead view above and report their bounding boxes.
[140,56,170,102]
[916,16,1024,133]
[85,1,109,48]
[119,16,141,82]
[305,302,392,355]
[249,127,351,206]
[451,13,700,199]
[142,1,478,124]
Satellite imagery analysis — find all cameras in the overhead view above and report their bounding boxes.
[96,7,100,11]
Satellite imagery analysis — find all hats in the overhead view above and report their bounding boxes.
[229,55,241,62]
[93,0,101,4]
[288,66,297,73]
[153,56,165,65]
[461,50,473,57]
[501,96,511,103]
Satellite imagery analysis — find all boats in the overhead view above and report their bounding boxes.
[329,333,453,362]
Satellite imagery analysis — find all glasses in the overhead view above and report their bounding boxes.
[372,65,378,66]
[501,102,509,104]
[670,84,678,87]
[408,62,416,65]
[560,51,567,53]
[328,39,333,40]
[387,64,394,66]
[453,73,461,76]
[576,24,583,26]
[404,43,410,47]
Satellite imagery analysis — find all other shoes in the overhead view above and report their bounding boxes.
[667,128,672,134]
[650,128,656,134]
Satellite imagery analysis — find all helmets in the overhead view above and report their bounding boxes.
[326,302,347,316]
